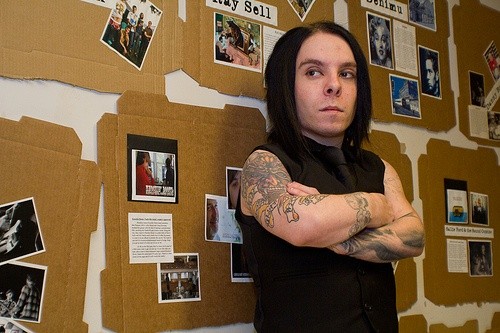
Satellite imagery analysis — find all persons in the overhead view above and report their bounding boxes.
[206,199,220,240]
[237,20,425,333]
[0,274,39,321]
[103,2,153,68]
[136,152,155,196]
[227,169,242,208]
[473,196,488,224]
[368,14,392,66]
[421,50,440,96]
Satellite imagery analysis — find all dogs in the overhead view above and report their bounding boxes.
[226,20,244,49]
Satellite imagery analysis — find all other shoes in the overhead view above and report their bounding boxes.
[129,47,132,52]
[134,52,138,58]
